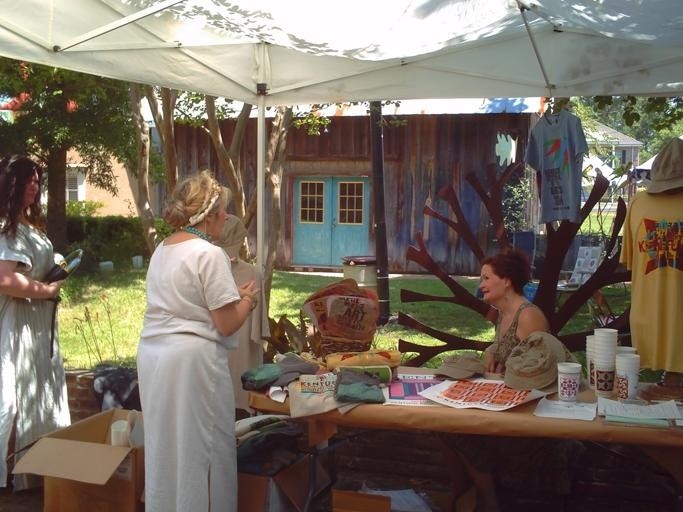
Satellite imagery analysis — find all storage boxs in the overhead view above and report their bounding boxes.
[4,408,145,512]
[236,428,476,512]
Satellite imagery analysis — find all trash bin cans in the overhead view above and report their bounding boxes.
[342,257,378,298]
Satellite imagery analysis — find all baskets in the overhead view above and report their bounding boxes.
[320,335,371,363]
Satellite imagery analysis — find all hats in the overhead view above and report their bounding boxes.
[504,332,565,390]
[646,137,683,194]
[431,355,484,380]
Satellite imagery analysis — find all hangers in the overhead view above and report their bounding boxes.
[543,102,560,118]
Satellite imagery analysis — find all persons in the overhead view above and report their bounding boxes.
[134,173,259,511]
[438,248,557,511]
[1,152,67,496]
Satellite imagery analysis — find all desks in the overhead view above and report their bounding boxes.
[248,363,683,511]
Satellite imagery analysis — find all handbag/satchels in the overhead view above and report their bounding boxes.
[241,357,317,389]
[334,372,385,403]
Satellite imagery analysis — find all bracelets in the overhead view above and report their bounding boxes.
[242,293,256,310]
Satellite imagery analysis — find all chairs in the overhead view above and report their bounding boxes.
[476,230,603,316]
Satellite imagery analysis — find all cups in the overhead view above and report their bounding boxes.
[558,328,640,402]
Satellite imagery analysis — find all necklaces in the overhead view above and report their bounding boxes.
[183,226,209,240]
